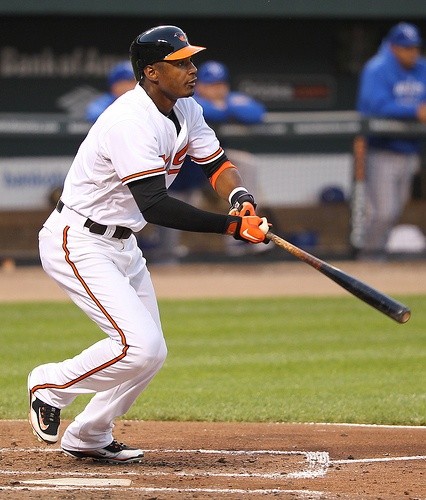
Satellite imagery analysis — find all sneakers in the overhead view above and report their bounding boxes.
[28,370,60,444]
[62,439,145,464]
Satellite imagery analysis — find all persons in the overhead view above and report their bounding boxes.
[25,26,273,465]
[86,59,139,125]
[348,22,426,260]
[167,60,266,258]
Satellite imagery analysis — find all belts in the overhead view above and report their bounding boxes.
[56,200,133,239]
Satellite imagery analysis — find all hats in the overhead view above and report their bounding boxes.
[107,64,134,87]
[195,61,228,82]
[385,22,419,46]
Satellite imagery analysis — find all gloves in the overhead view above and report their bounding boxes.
[224,215,273,244]
[227,187,257,217]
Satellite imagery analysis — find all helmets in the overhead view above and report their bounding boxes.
[129,25,206,80]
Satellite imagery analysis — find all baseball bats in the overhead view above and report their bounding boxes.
[231,209,412,322]
